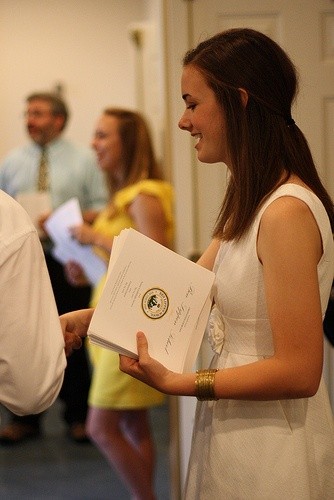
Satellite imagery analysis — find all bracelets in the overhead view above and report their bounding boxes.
[195,368,219,402]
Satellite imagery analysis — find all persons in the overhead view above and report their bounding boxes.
[68,109,176,500]
[0,92,108,447]
[0,189,68,418]
[60,28,334,500]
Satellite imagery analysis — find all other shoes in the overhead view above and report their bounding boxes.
[67,424,88,441]
[0,422,29,444]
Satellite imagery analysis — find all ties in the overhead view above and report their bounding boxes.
[37,143,49,191]
